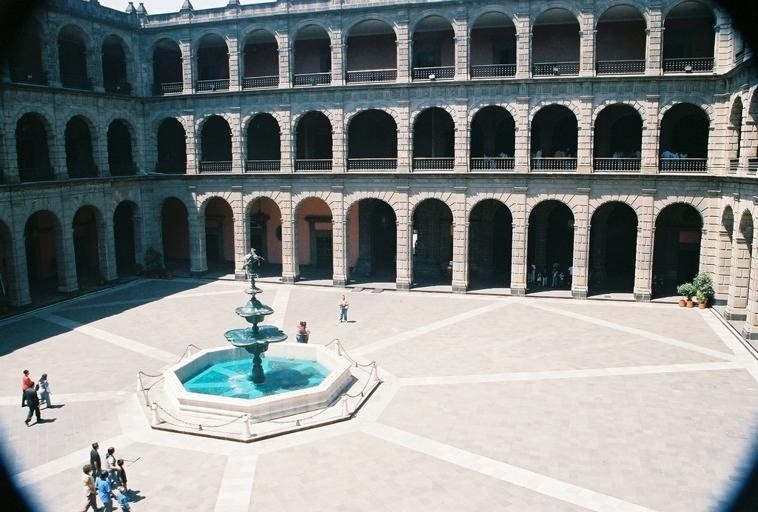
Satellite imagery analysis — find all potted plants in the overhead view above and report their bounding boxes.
[677,272,715,309]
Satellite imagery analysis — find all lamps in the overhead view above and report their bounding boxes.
[553,67,559,74]
[210,84,215,89]
[685,65,692,72]
[310,77,316,85]
[428,74,435,80]
[27,74,33,79]
[116,84,120,90]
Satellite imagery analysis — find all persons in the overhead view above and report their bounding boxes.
[528,263,559,288]
[82,443,128,512]
[21,370,34,407]
[295,321,311,343]
[24,381,44,425]
[338,295,349,323]
[38,374,52,407]
